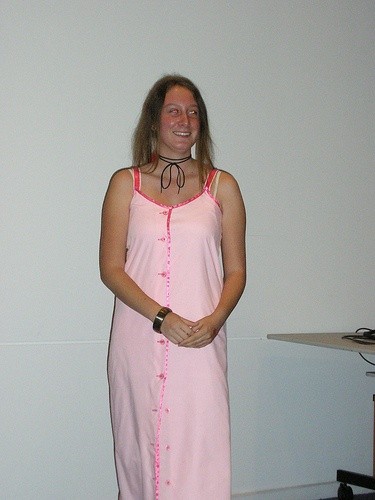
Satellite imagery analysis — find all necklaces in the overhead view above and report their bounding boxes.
[158,154,192,194]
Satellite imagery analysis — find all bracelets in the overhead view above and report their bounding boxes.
[153,307,172,334]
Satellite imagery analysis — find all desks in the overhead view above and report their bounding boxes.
[267,333,375,500]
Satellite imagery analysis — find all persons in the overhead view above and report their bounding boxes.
[99,74,246,500]
